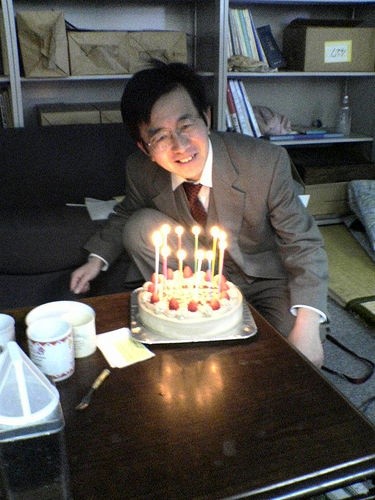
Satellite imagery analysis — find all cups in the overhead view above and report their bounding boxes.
[60,312,95,358]
[26,317,74,382]
[0,314,16,353]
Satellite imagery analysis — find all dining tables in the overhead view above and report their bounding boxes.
[0,290,375,500]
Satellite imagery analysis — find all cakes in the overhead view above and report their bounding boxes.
[138,266,243,338]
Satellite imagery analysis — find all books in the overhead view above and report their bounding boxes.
[227,8,268,68]
[267,126,344,141]
[226,80,262,138]
[257,25,285,68]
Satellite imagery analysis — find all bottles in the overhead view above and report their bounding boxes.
[335,95,352,136]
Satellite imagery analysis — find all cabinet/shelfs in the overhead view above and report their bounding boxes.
[0,0,375,225]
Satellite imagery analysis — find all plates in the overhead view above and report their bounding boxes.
[25,301,95,327]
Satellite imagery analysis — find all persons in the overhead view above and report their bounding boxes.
[71,62,329,369]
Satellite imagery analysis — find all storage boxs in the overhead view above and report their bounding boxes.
[282,18,375,72]
[305,164,375,216]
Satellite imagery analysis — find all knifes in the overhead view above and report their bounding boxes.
[76,369,111,410]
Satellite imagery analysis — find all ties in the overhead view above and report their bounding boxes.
[182,181,207,229]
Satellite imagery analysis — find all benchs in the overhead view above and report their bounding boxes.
[0,123,142,312]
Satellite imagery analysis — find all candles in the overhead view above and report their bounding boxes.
[150,225,229,293]
